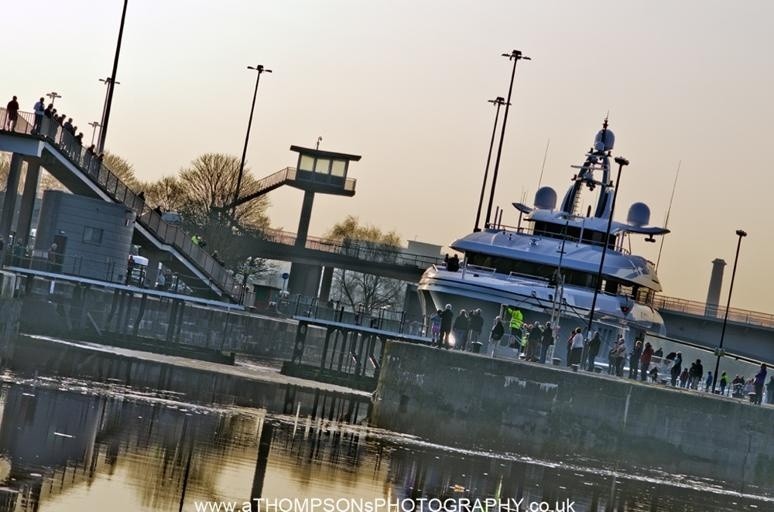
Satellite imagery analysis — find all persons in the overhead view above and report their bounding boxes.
[33,98,219,260]
[128,255,134,282]
[431,304,774,405]
[7,96,19,132]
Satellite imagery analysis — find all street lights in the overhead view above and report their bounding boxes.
[227,64,272,231]
[44,75,120,154]
[472,97,512,232]
[484,47,532,228]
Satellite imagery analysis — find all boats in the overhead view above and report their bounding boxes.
[419,109,673,380]
